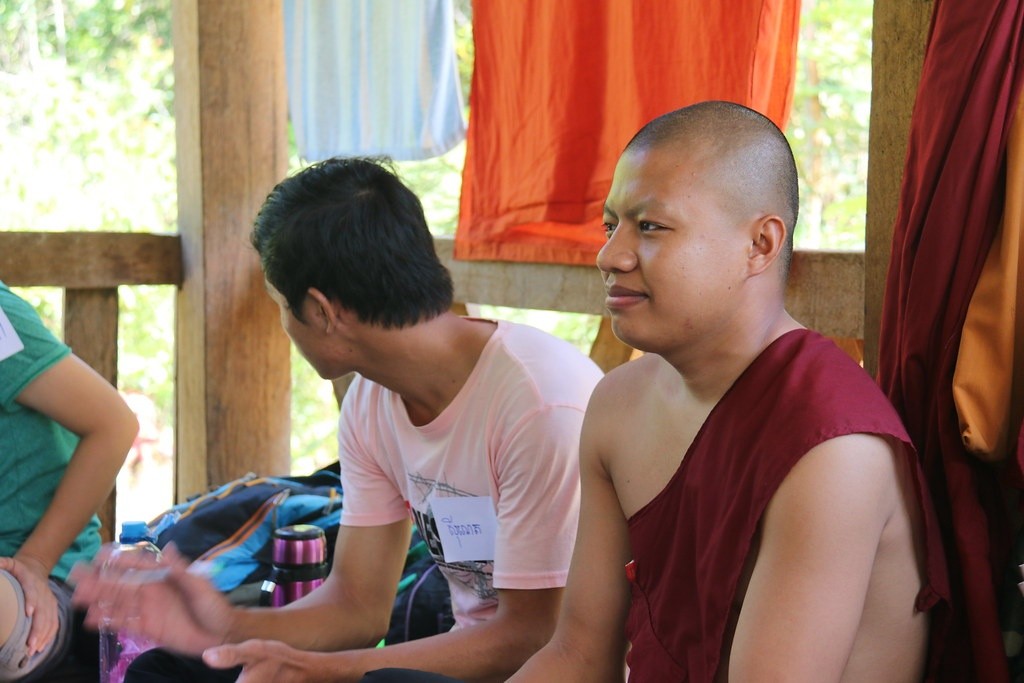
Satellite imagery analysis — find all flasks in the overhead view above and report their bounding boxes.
[272,524,329,607]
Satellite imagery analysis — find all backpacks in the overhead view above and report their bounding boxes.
[153,471,344,593]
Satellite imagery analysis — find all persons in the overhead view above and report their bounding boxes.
[68,156,604,682]
[379,101,954,683]
[0,280,140,680]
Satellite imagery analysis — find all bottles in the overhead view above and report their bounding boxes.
[100,521,170,683]
